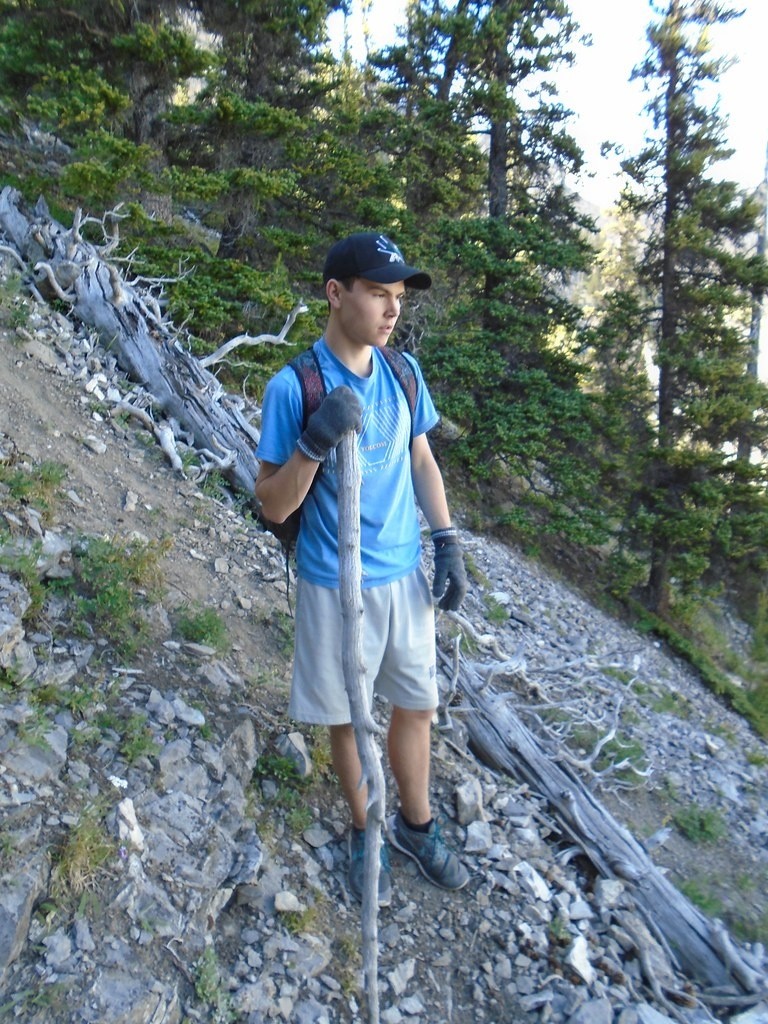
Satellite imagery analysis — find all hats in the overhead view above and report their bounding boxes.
[323,232,432,291]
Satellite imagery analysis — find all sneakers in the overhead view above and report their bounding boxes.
[387,809,469,891]
[347,826,391,906]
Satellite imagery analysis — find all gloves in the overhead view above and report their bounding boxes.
[431,528,466,611]
[296,385,362,462]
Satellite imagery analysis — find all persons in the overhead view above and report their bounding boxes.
[254,235,471,907]
